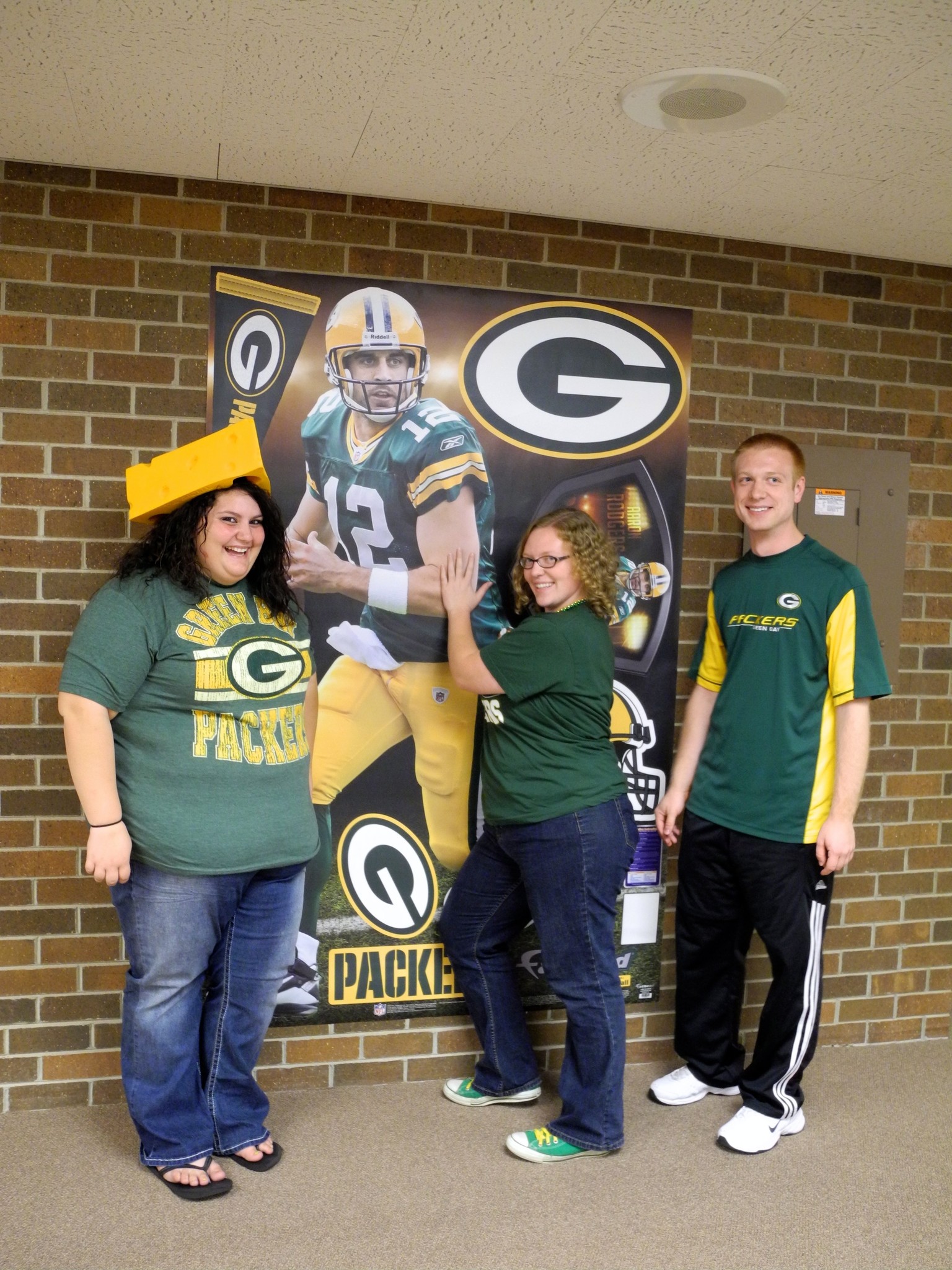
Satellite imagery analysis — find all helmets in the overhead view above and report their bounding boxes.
[324,285,430,397]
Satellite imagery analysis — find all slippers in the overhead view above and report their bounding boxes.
[228,1135,283,1172]
[144,1153,233,1200]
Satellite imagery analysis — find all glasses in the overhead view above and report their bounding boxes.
[519,553,576,569]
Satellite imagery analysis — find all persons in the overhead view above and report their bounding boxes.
[649,433,893,1156]
[271,291,503,1015]
[57,479,321,1198]
[607,556,671,626]
[437,507,642,1163]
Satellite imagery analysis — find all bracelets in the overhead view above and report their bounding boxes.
[90,819,122,827]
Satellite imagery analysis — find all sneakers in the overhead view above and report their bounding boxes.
[505,1126,610,1164]
[649,1060,743,1106]
[717,1101,805,1153]
[442,1076,542,1108]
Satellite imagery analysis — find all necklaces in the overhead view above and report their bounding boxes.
[555,599,586,613]
